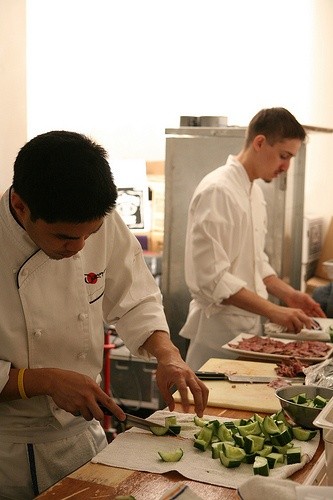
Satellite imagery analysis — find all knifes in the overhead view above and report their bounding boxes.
[97,403,176,437]
[193,370,292,384]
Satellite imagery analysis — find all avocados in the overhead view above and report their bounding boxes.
[148,393,328,477]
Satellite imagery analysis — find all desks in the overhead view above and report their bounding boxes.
[33,356,333,500]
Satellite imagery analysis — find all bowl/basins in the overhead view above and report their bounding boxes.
[274,386,333,431]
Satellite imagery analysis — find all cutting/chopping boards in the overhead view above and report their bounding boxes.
[171,357,304,415]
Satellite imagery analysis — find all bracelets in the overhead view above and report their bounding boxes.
[18,369,30,398]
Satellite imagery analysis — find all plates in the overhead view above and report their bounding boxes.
[221,332,333,364]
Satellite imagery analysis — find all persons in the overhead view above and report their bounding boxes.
[1,131,209,500]
[186,107,325,369]
